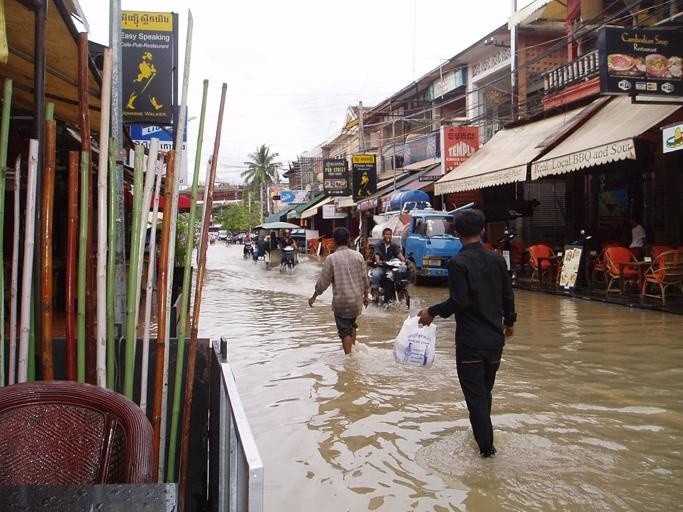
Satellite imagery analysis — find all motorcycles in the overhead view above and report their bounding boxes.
[363,255,413,315]
[540,228,593,292]
[203,228,305,274]
[489,233,520,288]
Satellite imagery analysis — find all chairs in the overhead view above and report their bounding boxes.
[529,235,682,309]
[309,234,336,256]
[0,379,157,486]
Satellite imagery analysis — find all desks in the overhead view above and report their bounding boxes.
[0,482,178,512]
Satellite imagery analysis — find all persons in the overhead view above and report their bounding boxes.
[629,216,648,261]
[418,208,516,457]
[308,226,372,354]
[371,228,410,301]
[244,231,254,260]
[269,231,276,249]
[276,229,298,273]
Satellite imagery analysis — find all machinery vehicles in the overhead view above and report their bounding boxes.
[367,198,485,286]
